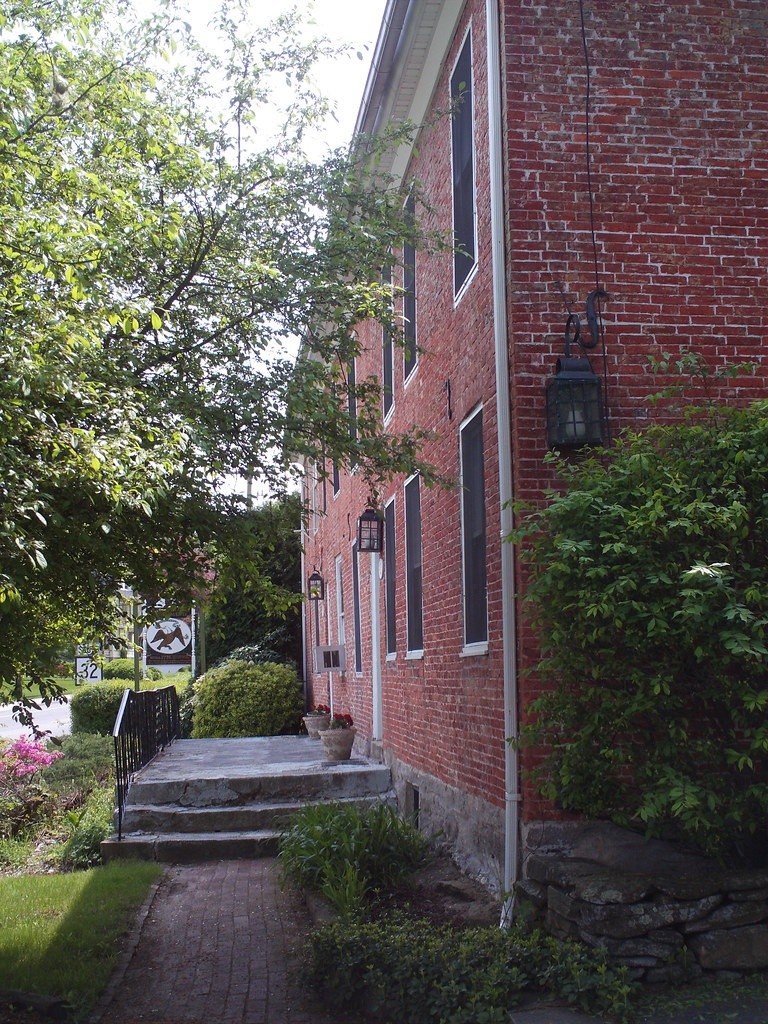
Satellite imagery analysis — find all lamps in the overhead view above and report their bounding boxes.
[308,566,324,601]
[542,286,611,460]
[355,509,383,554]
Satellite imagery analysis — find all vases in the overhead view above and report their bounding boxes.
[318,731,357,760]
[304,717,330,739]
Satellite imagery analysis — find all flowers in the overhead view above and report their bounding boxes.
[307,705,330,717]
[327,713,354,730]
